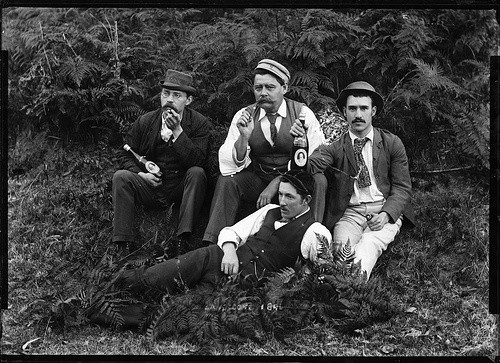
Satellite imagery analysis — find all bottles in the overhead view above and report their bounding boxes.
[122,144,163,181]
[290,113,309,173]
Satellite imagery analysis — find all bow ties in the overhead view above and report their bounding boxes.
[278,218,292,224]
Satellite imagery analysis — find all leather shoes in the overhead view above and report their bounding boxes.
[173,239,188,257]
[121,241,136,255]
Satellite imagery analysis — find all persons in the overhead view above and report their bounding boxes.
[203,59,328,243]
[89,169,334,329]
[109,69,212,256]
[290,79,413,284]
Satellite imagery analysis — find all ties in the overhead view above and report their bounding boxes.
[266,114,281,143]
[354,139,372,189]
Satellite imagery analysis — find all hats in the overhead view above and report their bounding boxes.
[158,69,196,94]
[255,59,290,84]
[335,80,385,114]
[283,168,315,199]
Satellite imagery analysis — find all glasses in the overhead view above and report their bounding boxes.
[161,92,181,99]
